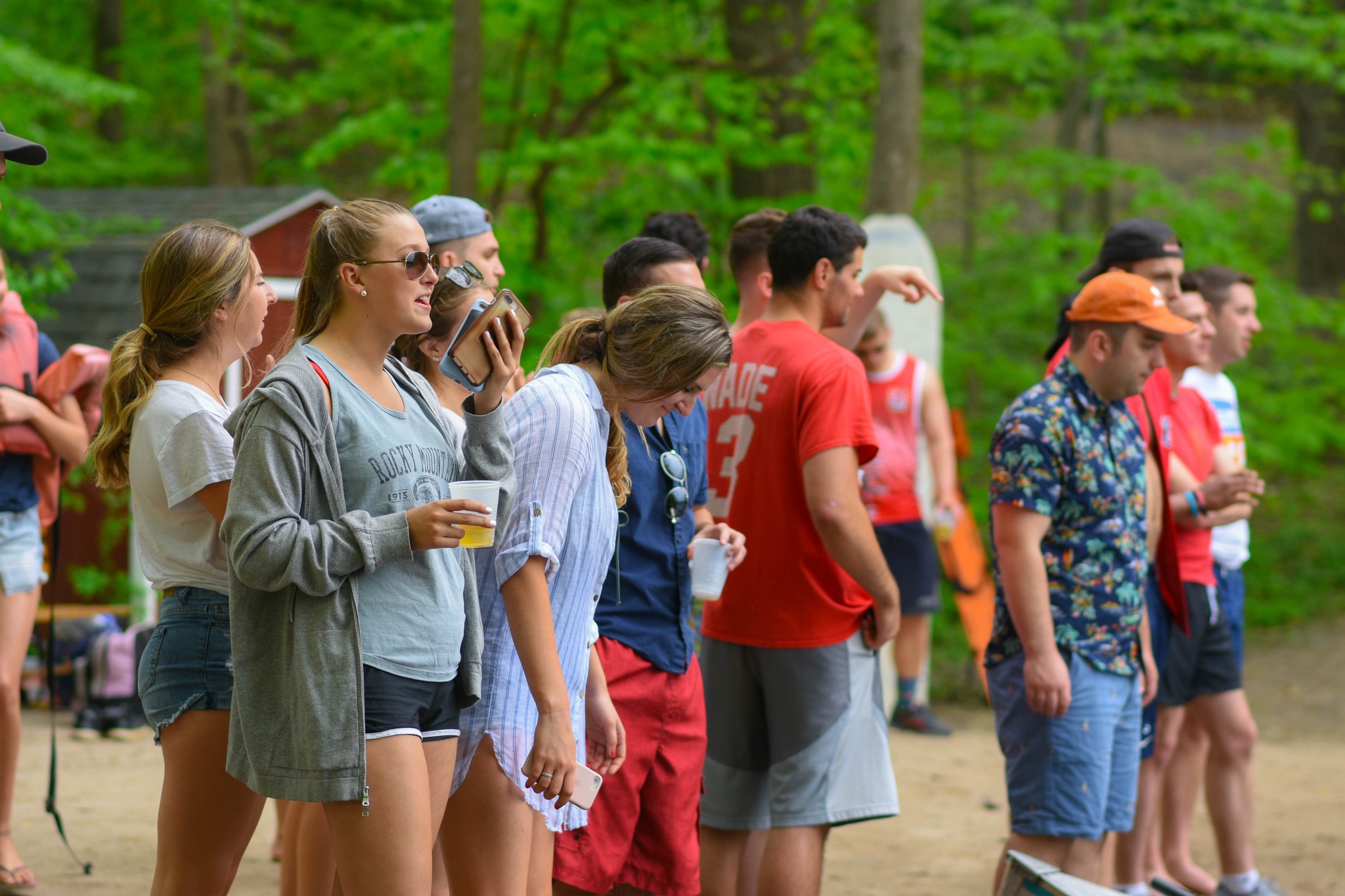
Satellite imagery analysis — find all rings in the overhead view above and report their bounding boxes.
[541,772,553,778]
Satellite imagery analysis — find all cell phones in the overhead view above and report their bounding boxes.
[439,289,532,393]
[520,743,603,810]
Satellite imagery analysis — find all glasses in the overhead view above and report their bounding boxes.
[430,260,485,305]
[334,251,441,281]
[661,449,688,523]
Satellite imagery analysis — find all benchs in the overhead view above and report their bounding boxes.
[31,601,129,706]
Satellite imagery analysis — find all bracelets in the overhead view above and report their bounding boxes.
[1185,491,1198,517]
[1195,488,1208,516]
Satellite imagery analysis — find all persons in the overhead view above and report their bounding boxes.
[0,125,1278,896]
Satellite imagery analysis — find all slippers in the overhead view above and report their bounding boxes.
[0,864,34,891]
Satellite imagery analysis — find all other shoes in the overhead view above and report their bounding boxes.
[889,704,955,736]
[1213,876,1286,896]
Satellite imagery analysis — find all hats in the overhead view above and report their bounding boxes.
[1065,266,1200,335]
[1076,219,1183,282]
[411,194,492,245]
[0,122,48,166]
[1040,291,1080,363]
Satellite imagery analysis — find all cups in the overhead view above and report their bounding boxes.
[447,479,501,548]
[1212,440,1244,475]
[690,538,734,601]
